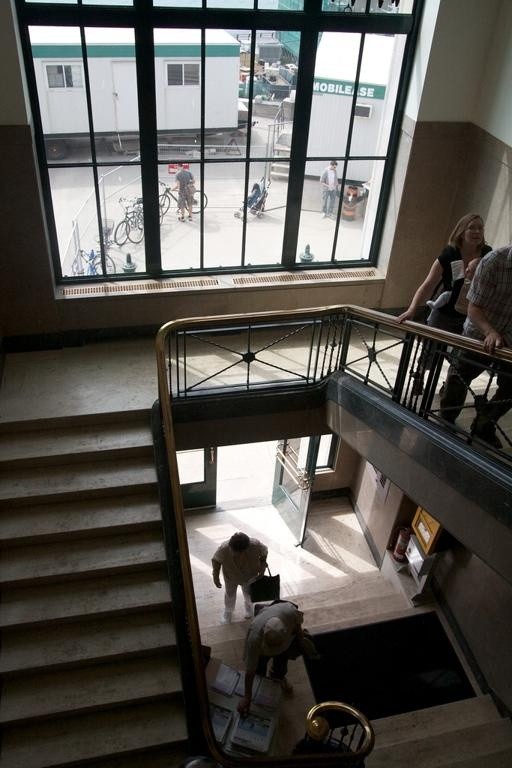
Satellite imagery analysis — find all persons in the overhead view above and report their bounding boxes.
[437,241,512,453]
[210,530,269,627]
[170,161,197,222]
[319,159,339,220]
[394,212,493,396]
[233,598,305,724]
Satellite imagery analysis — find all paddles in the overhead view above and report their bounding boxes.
[297,611,476,732]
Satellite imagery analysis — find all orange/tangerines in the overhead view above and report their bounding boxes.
[234,177,272,222]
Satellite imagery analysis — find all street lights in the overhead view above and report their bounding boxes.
[250,574,280,603]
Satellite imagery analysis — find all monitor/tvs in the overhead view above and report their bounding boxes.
[209,662,287,758]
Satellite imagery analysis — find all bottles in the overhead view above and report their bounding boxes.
[264,617,286,646]
[182,164,189,169]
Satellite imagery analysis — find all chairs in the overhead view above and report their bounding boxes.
[224,611,231,621]
[243,603,253,617]
[469,417,503,449]
[178,215,192,221]
[414,373,423,395]
[269,669,295,693]
[322,211,336,218]
